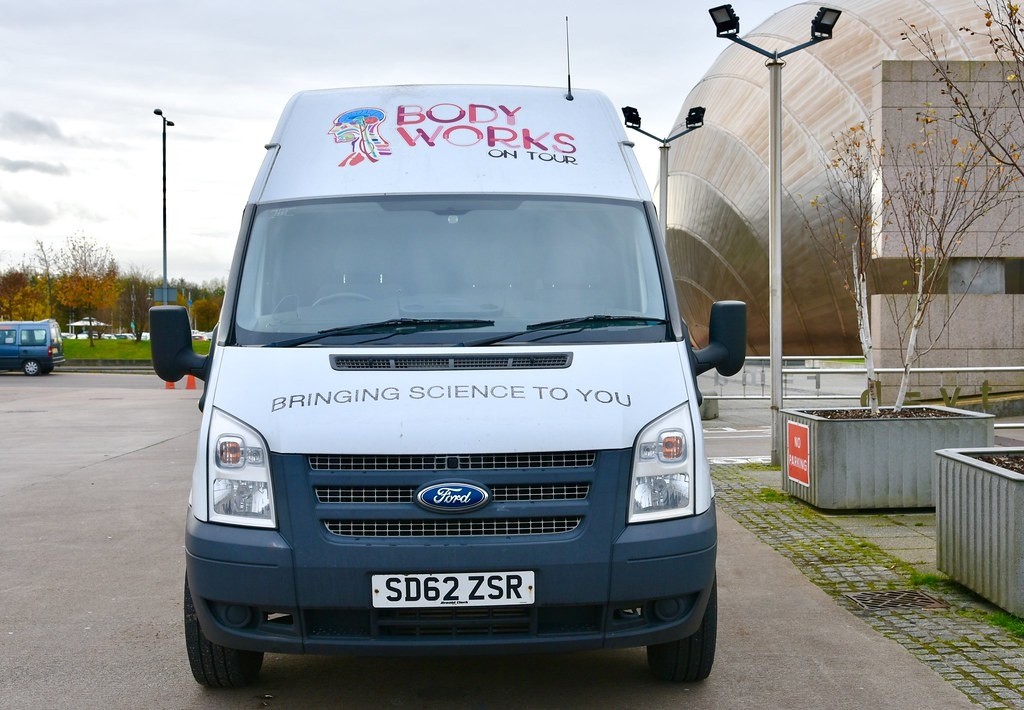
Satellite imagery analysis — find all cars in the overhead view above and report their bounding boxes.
[190,329,214,341]
[60,332,151,340]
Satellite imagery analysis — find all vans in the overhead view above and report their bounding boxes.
[147,80,720,693]
[0,319,66,376]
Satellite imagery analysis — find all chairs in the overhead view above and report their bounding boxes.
[309,228,614,307]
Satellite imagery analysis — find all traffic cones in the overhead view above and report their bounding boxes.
[165,381,176,389]
[185,375,196,390]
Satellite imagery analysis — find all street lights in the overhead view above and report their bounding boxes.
[153,108,175,303]
[620,104,708,254]
[708,2,842,471]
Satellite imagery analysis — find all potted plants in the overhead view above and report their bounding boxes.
[779,0,1024,621]
